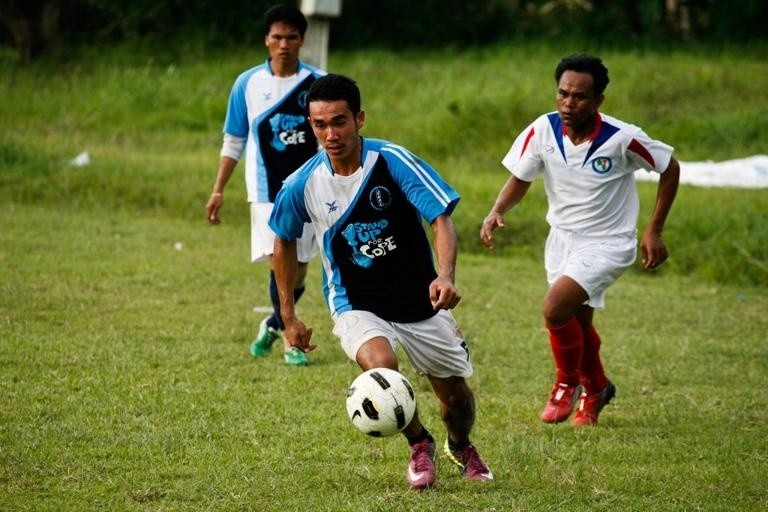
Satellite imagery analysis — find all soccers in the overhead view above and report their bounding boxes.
[346,368,416,437]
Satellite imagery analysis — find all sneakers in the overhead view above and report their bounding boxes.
[443,437,493,480]
[283,345,310,366]
[540,382,579,423]
[407,432,436,489]
[572,378,615,426]
[249,315,281,360]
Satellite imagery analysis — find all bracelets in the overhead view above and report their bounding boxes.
[208,191,224,197]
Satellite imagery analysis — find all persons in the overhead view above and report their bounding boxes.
[265,70,494,490]
[201,3,330,364]
[475,49,683,431]
